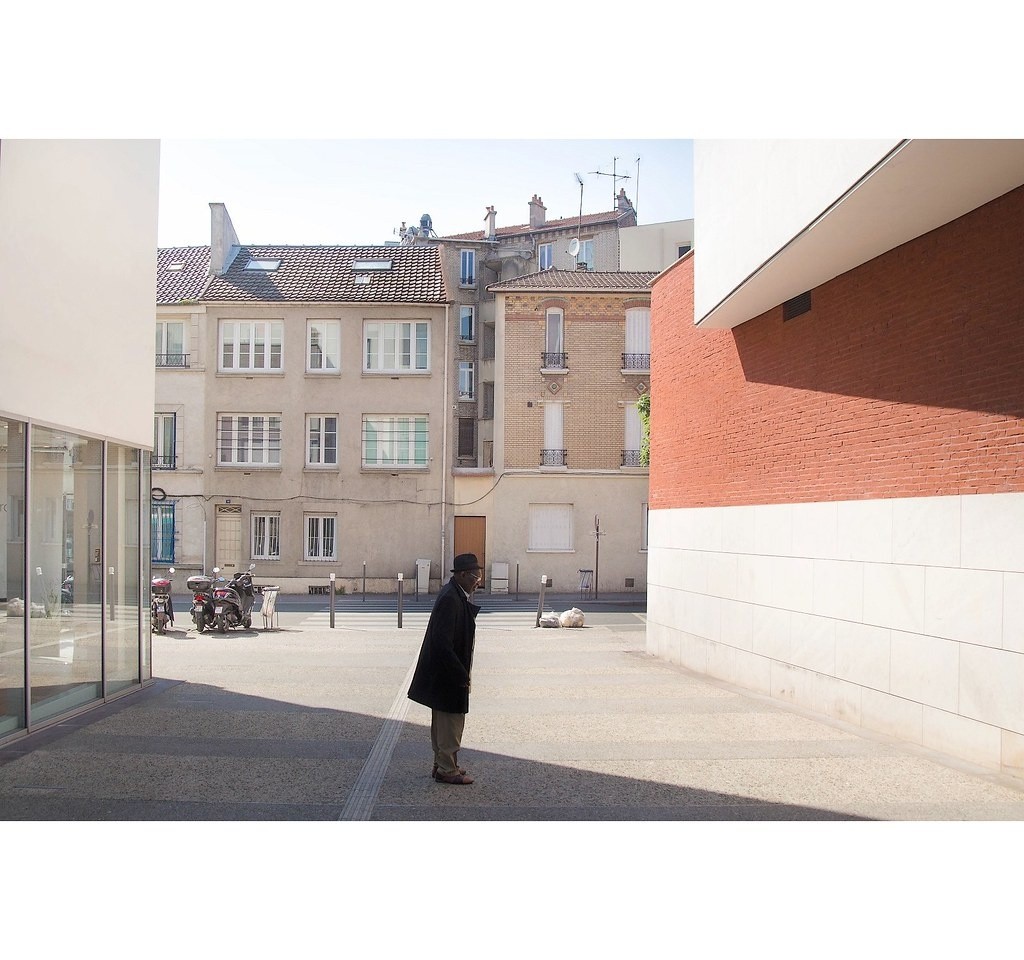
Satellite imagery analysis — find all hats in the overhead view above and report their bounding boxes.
[450,553,485,572]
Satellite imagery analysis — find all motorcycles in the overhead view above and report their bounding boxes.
[151,568,176,635]
[212,563,256,634]
[62,575,74,616]
[187,567,225,633]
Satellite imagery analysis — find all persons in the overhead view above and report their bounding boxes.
[407,553,483,784]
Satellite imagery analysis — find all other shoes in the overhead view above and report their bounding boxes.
[431,765,466,778]
[435,770,474,783]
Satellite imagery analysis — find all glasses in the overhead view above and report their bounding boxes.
[465,571,482,582]
